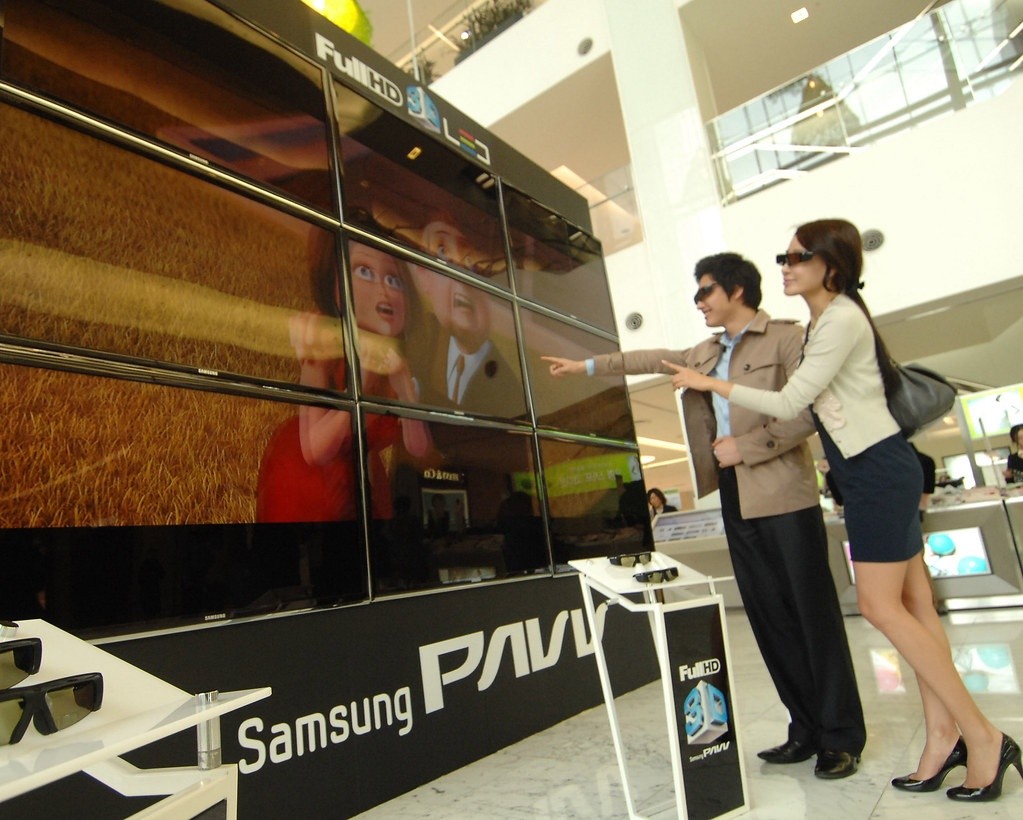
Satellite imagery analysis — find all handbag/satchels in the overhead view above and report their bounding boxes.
[881,358,957,434]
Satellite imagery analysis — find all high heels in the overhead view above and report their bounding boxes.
[891,735,967,793]
[946,733,1023,802]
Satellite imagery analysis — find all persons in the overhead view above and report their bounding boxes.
[1002,423,1023,484]
[662,218,1023,802]
[541,253,868,779]
[647,488,679,517]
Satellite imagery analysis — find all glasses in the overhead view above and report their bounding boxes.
[608,552,652,568]
[776,251,816,267]
[694,279,719,304]
[632,568,679,583]
[0,638,42,690]
[0,671,102,747]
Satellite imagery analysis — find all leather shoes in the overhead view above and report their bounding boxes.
[814,749,861,779]
[756,734,819,764]
